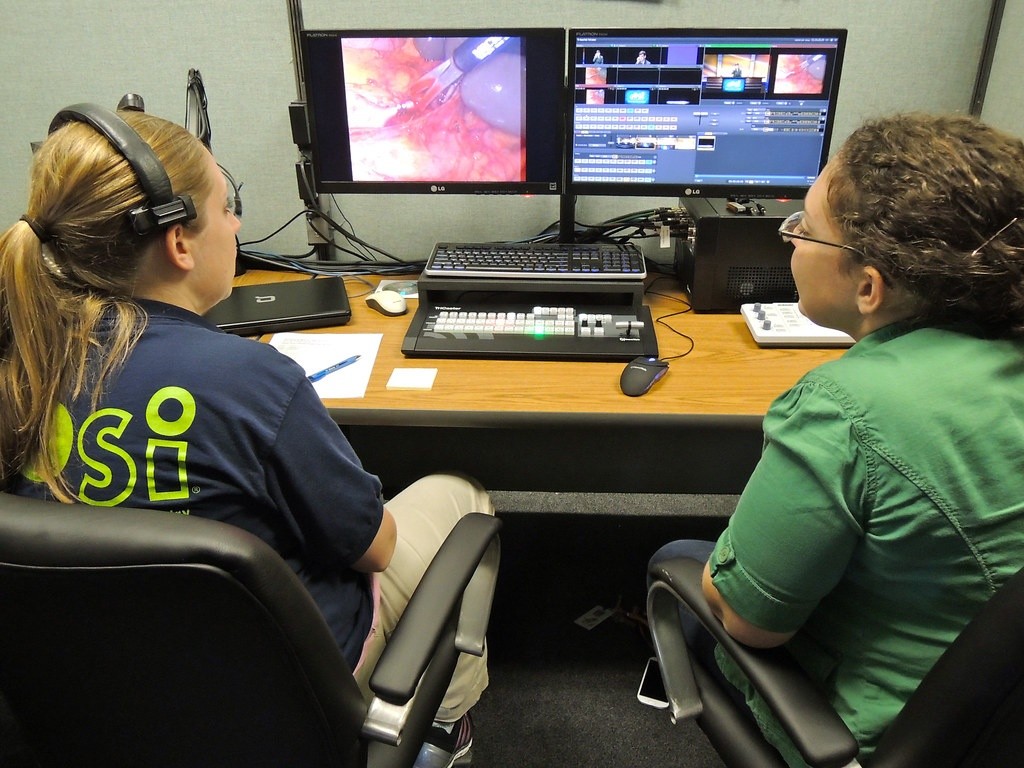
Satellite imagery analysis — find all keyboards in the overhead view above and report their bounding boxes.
[424,242,648,281]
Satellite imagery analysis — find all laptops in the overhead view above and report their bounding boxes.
[205,275,352,336]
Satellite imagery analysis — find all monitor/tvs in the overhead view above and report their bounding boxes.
[564,28,847,200]
[300,26,567,195]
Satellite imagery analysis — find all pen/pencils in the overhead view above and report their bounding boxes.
[308,355,361,382]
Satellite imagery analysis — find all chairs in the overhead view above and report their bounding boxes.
[0,489,504,768]
[645,557,1024,768]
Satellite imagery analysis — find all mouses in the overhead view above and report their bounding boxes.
[365,291,408,317]
[619,356,670,396]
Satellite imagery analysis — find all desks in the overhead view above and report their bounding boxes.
[233,268,853,529]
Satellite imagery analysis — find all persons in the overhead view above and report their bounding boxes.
[592,50,604,65]
[635,51,651,65]
[646,117,1024,763]
[731,63,742,78]
[0,109,498,767]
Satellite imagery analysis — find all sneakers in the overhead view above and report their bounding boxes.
[412,711,475,768]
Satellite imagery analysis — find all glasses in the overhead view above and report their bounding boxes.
[778,211,895,286]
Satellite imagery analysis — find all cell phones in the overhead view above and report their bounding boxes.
[637,657,670,709]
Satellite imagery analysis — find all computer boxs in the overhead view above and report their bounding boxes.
[672,197,805,313]
